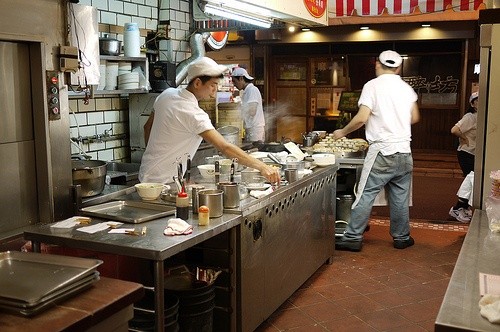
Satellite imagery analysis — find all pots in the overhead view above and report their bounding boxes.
[337,194,355,223]
[98,33,122,55]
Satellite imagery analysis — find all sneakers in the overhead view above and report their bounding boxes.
[449,206,472,223]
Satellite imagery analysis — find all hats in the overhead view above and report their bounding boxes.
[379,50,403,68]
[231,68,255,80]
[188,57,227,85]
[470,92,478,103]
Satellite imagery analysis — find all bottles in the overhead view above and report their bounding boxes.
[176,187,190,220]
[330,62,342,87]
[198,205,210,225]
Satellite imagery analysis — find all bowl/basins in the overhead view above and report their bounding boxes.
[71,156,107,197]
[196,164,222,178]
[123,23,141,57]
[135,183,165,200]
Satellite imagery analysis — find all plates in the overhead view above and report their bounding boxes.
[96,60,140,90]
[312,154,336,167]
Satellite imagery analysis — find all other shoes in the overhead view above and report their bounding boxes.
[335,238,361,252]
[394,236,414,249]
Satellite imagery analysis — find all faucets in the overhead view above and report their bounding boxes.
[104,127,113,139]
[280,135,292,145]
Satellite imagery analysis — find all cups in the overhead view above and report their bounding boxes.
[192,182,239,219]
[285,169,299,184]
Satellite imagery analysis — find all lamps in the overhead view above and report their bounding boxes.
[197,1,271,28]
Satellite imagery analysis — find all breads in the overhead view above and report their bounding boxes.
[318,133,359,149]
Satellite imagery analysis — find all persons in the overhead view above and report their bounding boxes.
[448,91,479,223]
[231,68,266,142]
[138,56,280,185]
[330,50,420,251]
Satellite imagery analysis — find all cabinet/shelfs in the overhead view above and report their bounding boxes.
[69,54,150,99]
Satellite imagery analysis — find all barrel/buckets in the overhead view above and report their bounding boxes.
[129,282,216,332]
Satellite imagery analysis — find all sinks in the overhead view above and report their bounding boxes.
[105,161,141,180]
[260,144,286,153]
